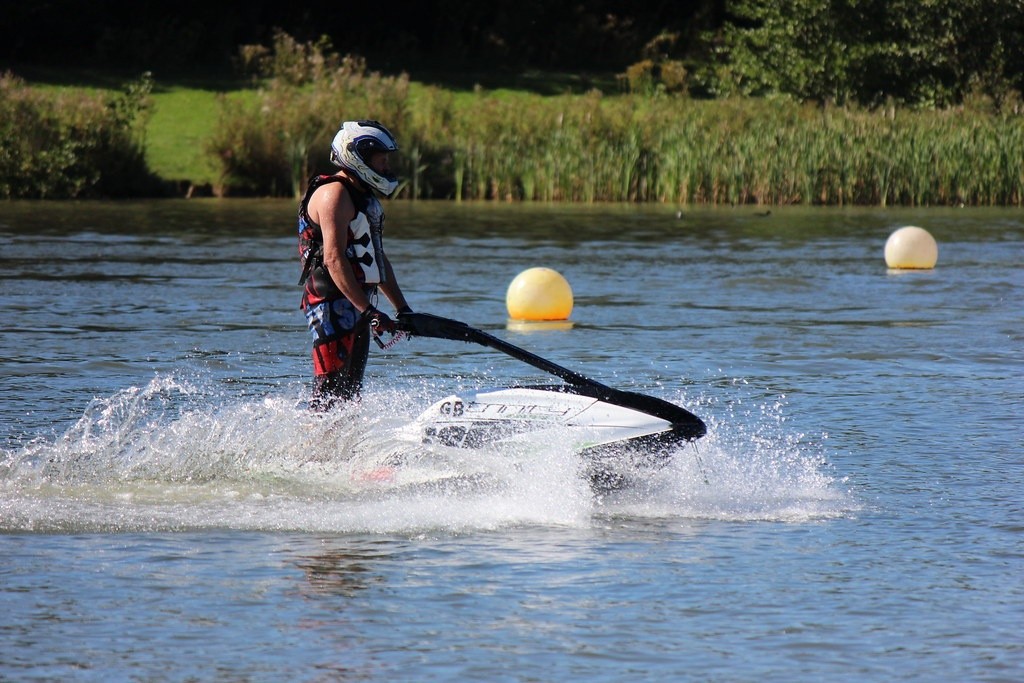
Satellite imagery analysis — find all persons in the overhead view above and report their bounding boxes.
[296,119,413,415]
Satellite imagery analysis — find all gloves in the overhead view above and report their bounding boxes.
[360,303,396,338]
[396,305,415,339]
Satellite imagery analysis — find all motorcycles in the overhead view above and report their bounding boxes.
[293,309,707,486]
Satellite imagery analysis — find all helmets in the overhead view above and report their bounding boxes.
[328,118,400,195]
